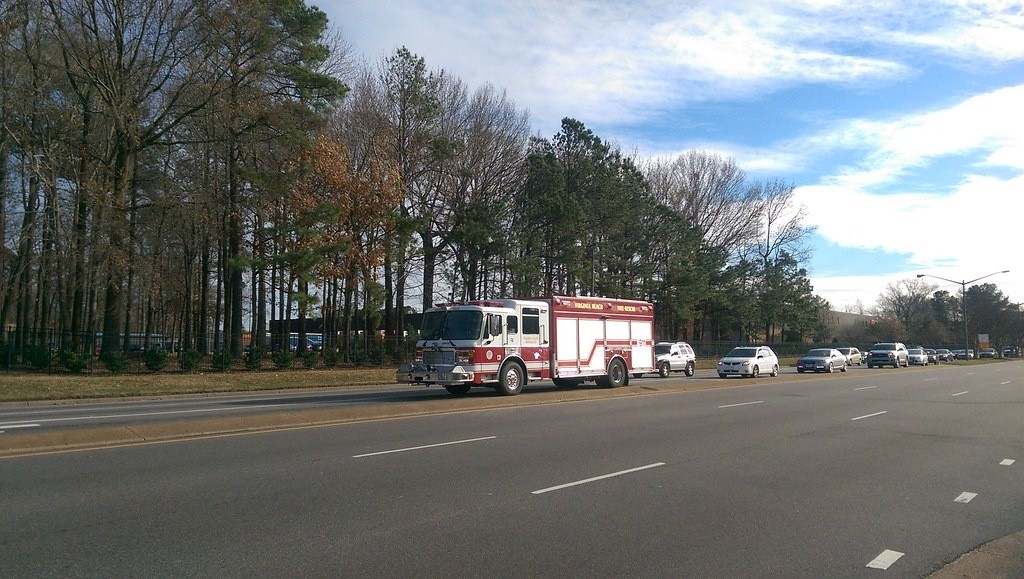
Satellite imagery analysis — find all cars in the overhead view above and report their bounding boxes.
[837,347,868,366]
[1003,345,1022,357]
[907,349,929,366]
[979,348,999,359]
[924,349,975,365]
[796,348,847,373]
[716,346,779,378]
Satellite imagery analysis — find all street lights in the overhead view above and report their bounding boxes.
[917,269,1010,361]
[590,234,646,297]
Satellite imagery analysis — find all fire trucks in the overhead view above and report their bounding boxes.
[395,296,659,396]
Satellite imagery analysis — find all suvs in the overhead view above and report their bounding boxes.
[633,340,697,378]
[867,341,909,368]
